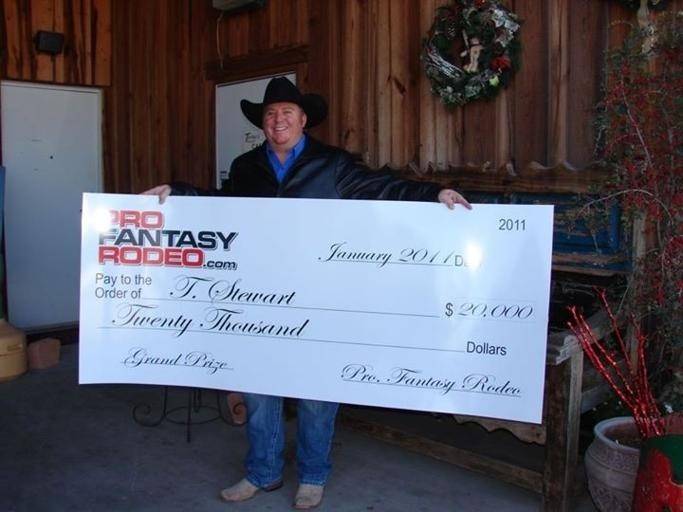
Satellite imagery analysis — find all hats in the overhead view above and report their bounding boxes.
[238,76,329,132]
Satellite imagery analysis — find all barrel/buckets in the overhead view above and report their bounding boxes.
[0,317,30,382]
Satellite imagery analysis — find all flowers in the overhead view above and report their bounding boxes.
[415,2,524,108]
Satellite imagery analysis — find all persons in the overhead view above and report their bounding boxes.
[137,73,474,509]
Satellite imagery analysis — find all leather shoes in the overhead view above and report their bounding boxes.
[291,482,325,510]
[217,472,283,503]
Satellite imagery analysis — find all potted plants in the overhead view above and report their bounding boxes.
[569,1,681,512]
